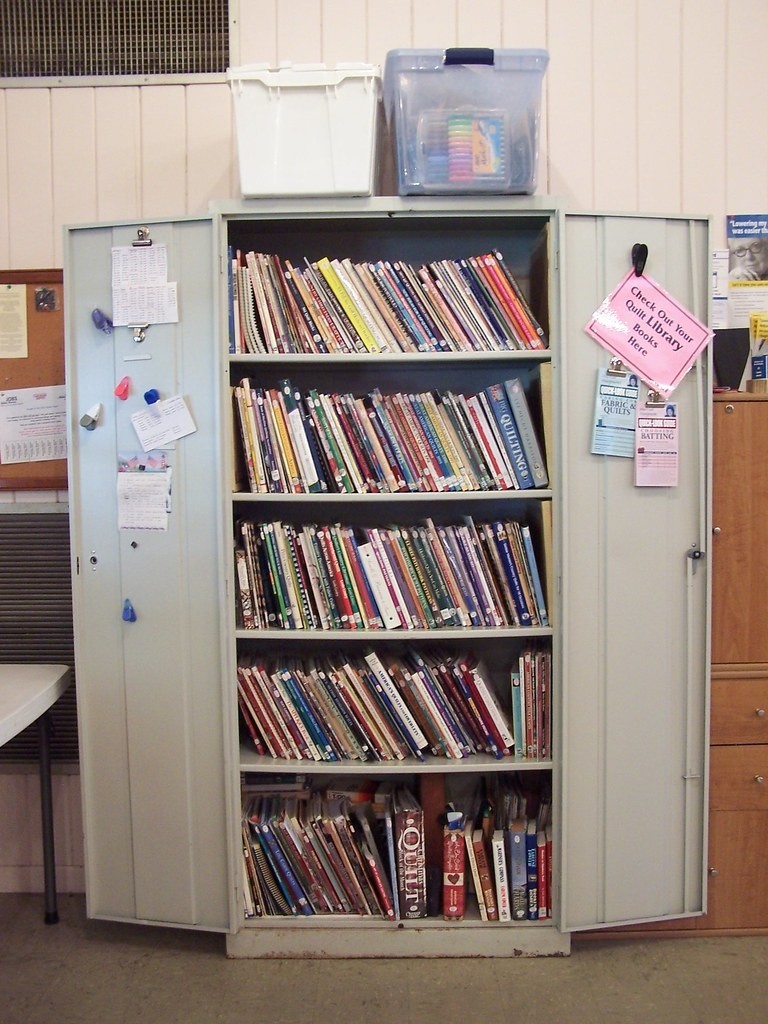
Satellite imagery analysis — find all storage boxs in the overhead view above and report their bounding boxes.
[224,62,385,203]
[383,44,553,198]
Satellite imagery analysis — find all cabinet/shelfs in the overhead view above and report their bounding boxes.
[55,210,712,952]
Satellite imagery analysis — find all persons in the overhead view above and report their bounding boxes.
[731,236,767,282]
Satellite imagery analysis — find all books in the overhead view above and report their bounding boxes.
[227,245,549,353]
[240,773,555,924]
[234,515,550,630]
[238,648,554,764]
[234,375,550,494]
